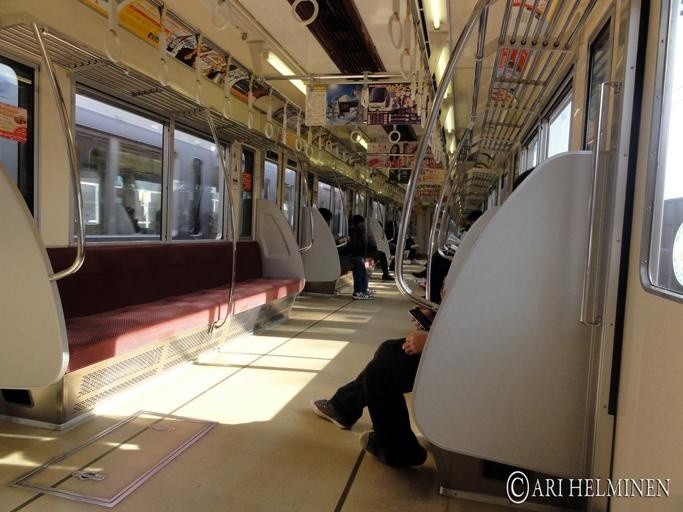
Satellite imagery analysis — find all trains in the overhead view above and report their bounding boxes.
[0,63,386,253]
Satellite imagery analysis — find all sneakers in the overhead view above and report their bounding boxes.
[382,275,395,280]
[352,288,375,300]
[360,430,427,465]
[311,397,352,429]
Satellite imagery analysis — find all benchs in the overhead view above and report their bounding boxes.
[0,147,585,505]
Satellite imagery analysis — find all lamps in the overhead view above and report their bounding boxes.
[430,0,454,154]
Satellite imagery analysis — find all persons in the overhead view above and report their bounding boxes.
[384,143,402,168]
[347,213,394,280]
[411,209,484,304]
[310,164,538,469]
[203,65,238,85]
[317,207,376,300]
[377,218,417,261]
[173,45,213,68]
